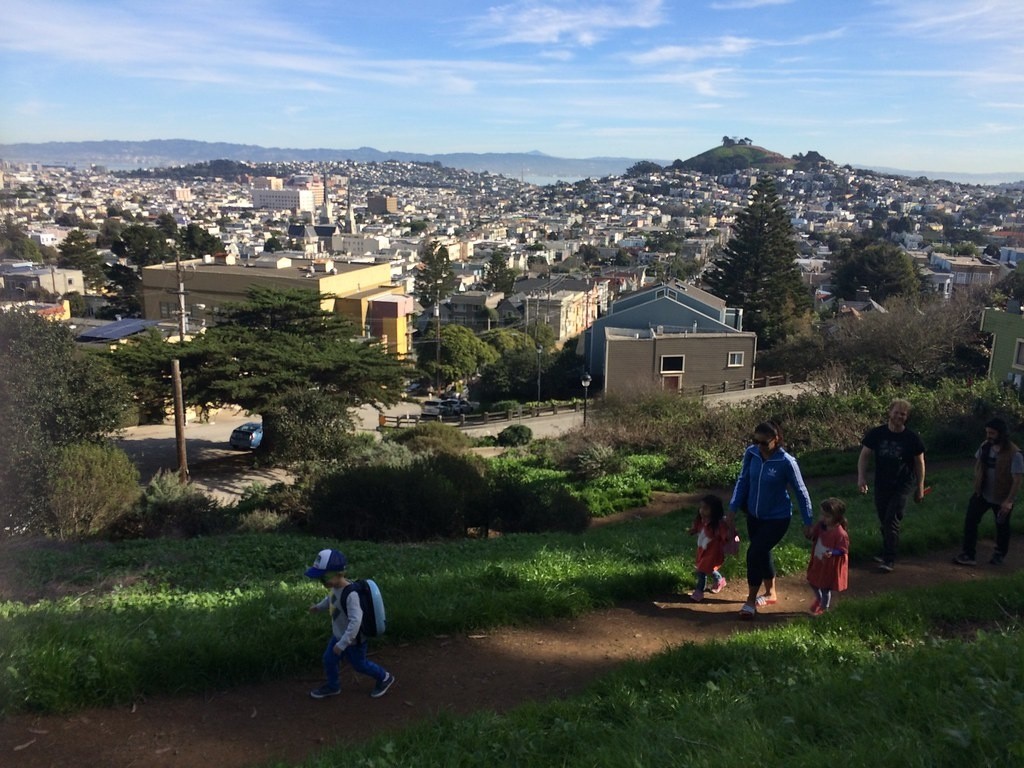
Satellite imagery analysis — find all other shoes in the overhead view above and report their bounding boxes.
[690,590,704,601]
[988,554,1005,566]
[810,600,824,615]
[710,578,727,594]
[955,553,977,566]
[871,554,894,572]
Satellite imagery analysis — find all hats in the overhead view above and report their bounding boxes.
[985,417,1007,432]
[304,549,346,578]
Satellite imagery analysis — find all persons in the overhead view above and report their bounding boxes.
[685,495,732,601]
[857,399,926,571]
[953,417,1024,566]
[802,498,850,615]
[725,420,813,617]
[304,548,394,698]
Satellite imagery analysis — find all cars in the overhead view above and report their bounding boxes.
[420,400,454,418]
[447,398,479,416]
[229,423,263,450]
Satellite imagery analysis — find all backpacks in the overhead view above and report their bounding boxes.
[714,520,739,555]
[341,579,385,637]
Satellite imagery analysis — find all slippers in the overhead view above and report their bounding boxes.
[739,605,757,619]
[755,596,777,607]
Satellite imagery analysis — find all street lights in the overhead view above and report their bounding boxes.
[580,371,593,427]
[177,304,207,343]
[536,345,544,417]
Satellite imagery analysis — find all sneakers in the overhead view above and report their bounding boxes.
[311,685,340,698]
[371,675,394,698]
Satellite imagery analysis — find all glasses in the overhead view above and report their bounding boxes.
[752,435,778,446]
[320,572,337,584]
